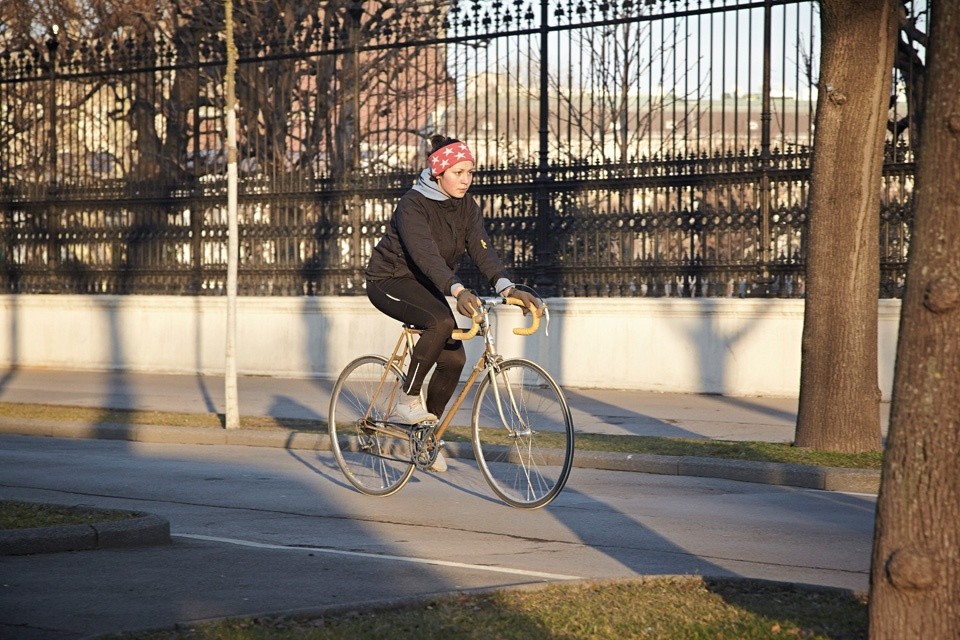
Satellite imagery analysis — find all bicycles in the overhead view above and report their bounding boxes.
[327,283,576,510]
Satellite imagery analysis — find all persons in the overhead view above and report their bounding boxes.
[366,136,546,473]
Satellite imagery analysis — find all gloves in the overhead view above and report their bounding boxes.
[457,289,478,318]
[507,287,539,316]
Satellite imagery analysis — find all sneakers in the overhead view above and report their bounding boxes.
[396,394,437,424]
[428,450,447,472]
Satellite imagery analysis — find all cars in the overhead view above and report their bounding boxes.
[2,143,414,183]
